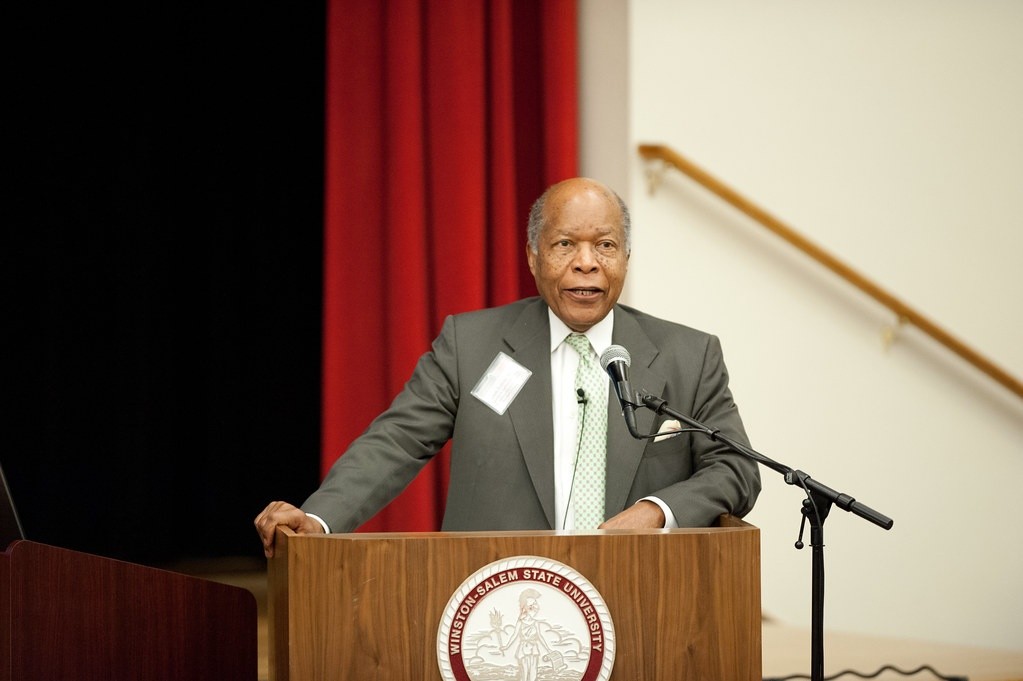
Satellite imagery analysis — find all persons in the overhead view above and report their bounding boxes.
[255,177,762,559]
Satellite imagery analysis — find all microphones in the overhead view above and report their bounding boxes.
[577,388,587,403]
[599,345,644,439]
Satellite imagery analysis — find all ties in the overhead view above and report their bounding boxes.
[564,335,608,529]
